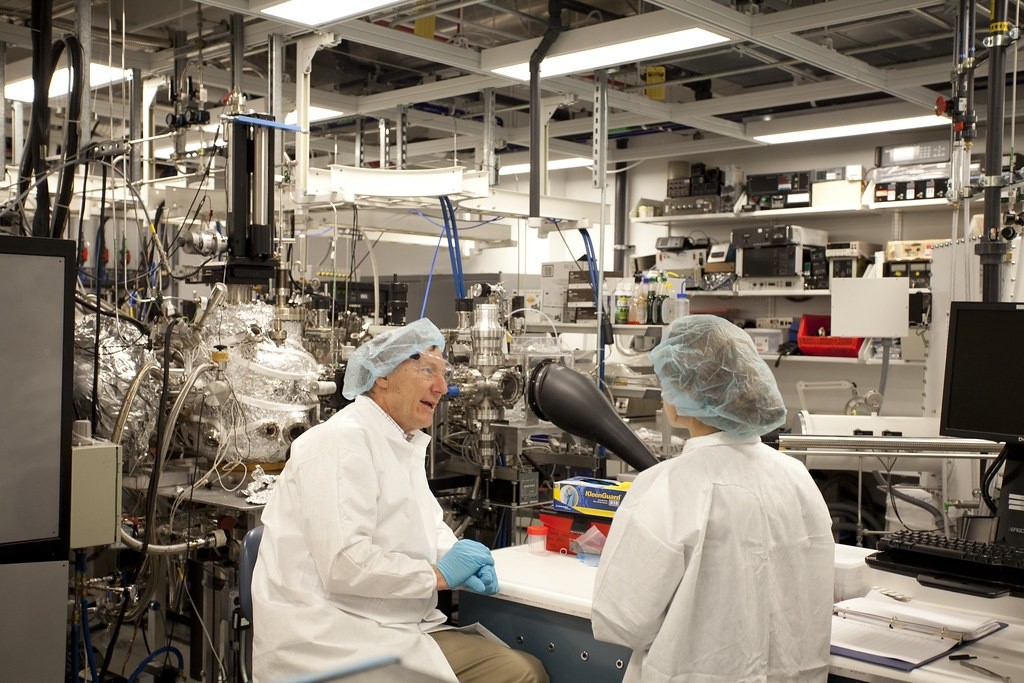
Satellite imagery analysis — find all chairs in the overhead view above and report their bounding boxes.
[239,527,265,683]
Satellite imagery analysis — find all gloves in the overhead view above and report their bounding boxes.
[459,564,499,595]
[434,538,494,590]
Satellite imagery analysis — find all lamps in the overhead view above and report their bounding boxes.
[499,151,594,174]
[745,101,953,144]
[481,9,747,83]
[155,97,359,159]
[4,40,127,102]
[249,0,412,29]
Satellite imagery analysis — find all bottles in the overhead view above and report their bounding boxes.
[674,293,690,318]
[619,275,674,325]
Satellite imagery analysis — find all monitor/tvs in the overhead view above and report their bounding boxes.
[939,301,1024,443]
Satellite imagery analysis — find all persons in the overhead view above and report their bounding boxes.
[588,309,837,683]
[247,312,551,683]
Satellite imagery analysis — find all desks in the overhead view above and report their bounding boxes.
[449,541,1024,683]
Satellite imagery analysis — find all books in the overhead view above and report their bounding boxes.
[827,584,1012,672]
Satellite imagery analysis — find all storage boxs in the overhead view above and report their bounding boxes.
[70,435,122,549]
[538,476,634,555]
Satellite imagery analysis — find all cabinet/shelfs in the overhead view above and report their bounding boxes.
[629,197,986,367]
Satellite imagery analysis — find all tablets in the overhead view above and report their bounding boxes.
[917,573,1011,598]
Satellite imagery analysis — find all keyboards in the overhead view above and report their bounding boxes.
[876,529,1024,573]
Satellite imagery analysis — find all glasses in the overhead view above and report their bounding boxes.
[398,361,453,383]
